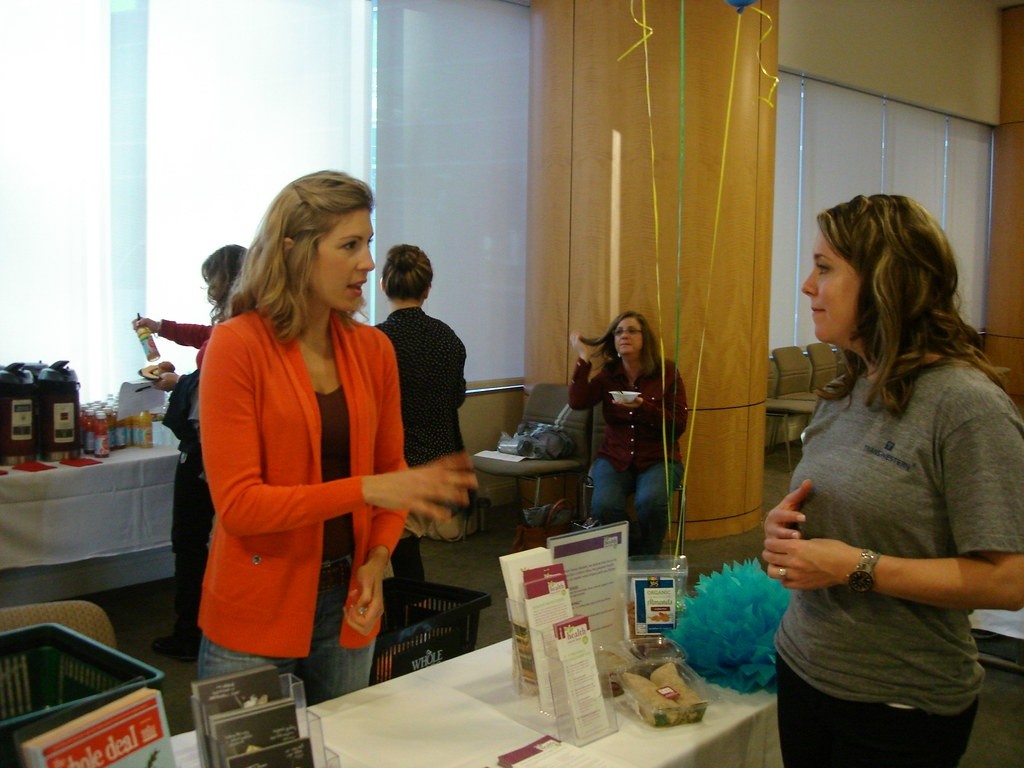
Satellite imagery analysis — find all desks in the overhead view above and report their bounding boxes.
[0,420,181,612]
[177,630,785,768]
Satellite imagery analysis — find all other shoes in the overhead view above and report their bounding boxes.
[153,634,199,661]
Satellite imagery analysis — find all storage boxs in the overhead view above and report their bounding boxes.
[0,621,168,767]
[368,574,492,689]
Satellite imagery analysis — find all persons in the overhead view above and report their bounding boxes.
[758,195,1024,768]
[367,244,469,608]
[197,169,477,710]
[132,245,249,653]
[567,311,690,560]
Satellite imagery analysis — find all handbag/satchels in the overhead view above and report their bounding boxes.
[425,499,479,544]
[510,500,575,553]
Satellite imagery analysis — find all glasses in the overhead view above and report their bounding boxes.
[612,327,642,337]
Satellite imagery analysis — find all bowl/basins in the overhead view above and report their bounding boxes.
[608,391,641,403]
[605,638,719,724]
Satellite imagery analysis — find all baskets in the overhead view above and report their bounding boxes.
[371,582,491,687]
[0,622,166,768]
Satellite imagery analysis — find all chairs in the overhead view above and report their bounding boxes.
[766,343,844,475]
[472,383,591,526]
[582,398,687,562]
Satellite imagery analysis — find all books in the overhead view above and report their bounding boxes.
[21,688,176,768]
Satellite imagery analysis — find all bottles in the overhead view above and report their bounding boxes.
[134,320,160,362]
[80,396,170,457]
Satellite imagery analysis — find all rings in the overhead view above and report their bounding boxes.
[779,567,788,581]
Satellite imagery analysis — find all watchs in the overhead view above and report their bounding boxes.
[846,548,881,596]
